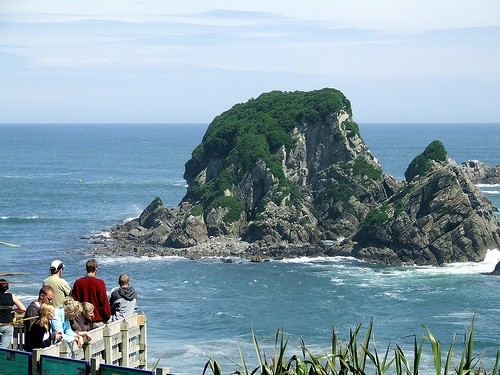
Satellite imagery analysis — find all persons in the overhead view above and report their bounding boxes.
[110,273,137,323]
[24,285,95,352]
[73,259,111,329]
[0,279,26,349]
[41,260,70,308]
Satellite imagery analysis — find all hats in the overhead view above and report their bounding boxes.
[50,259,65,273]
